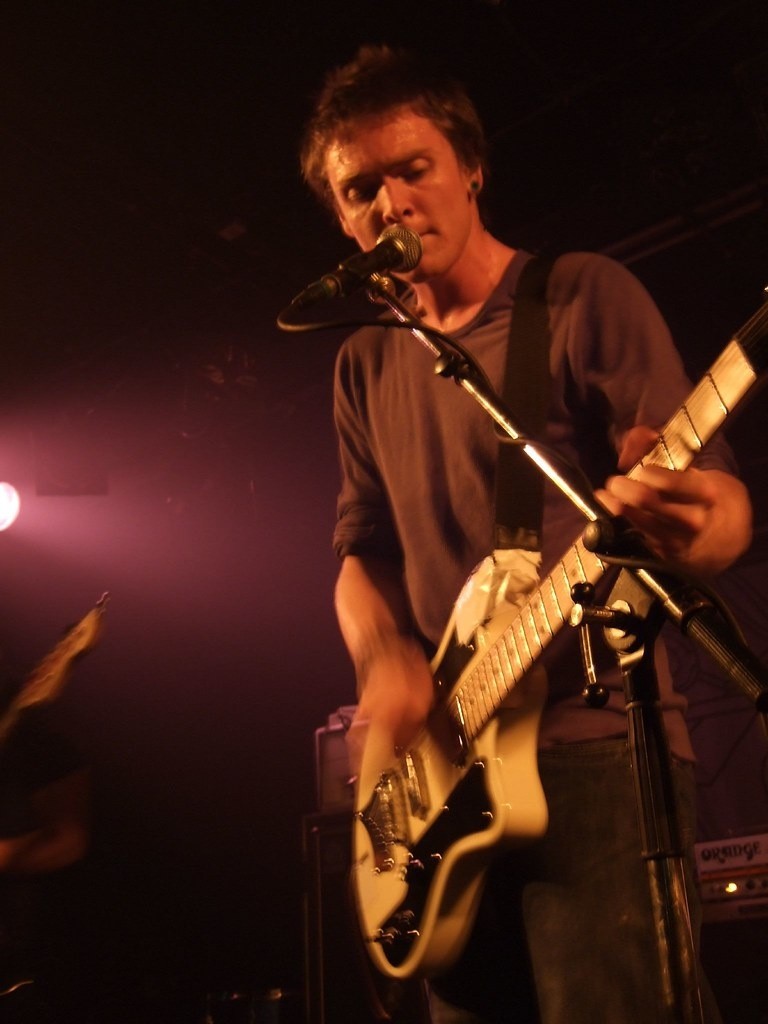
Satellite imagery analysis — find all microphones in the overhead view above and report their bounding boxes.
[292,225,423,311]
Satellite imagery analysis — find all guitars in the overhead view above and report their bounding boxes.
[0,586,117,741]
[351,304,768,985]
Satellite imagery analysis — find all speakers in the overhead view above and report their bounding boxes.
[698,897,768,1024]
[300,808,432,1024]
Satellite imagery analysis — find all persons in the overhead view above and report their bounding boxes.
[297,43,756,1024]
[0,684,98,1000]
